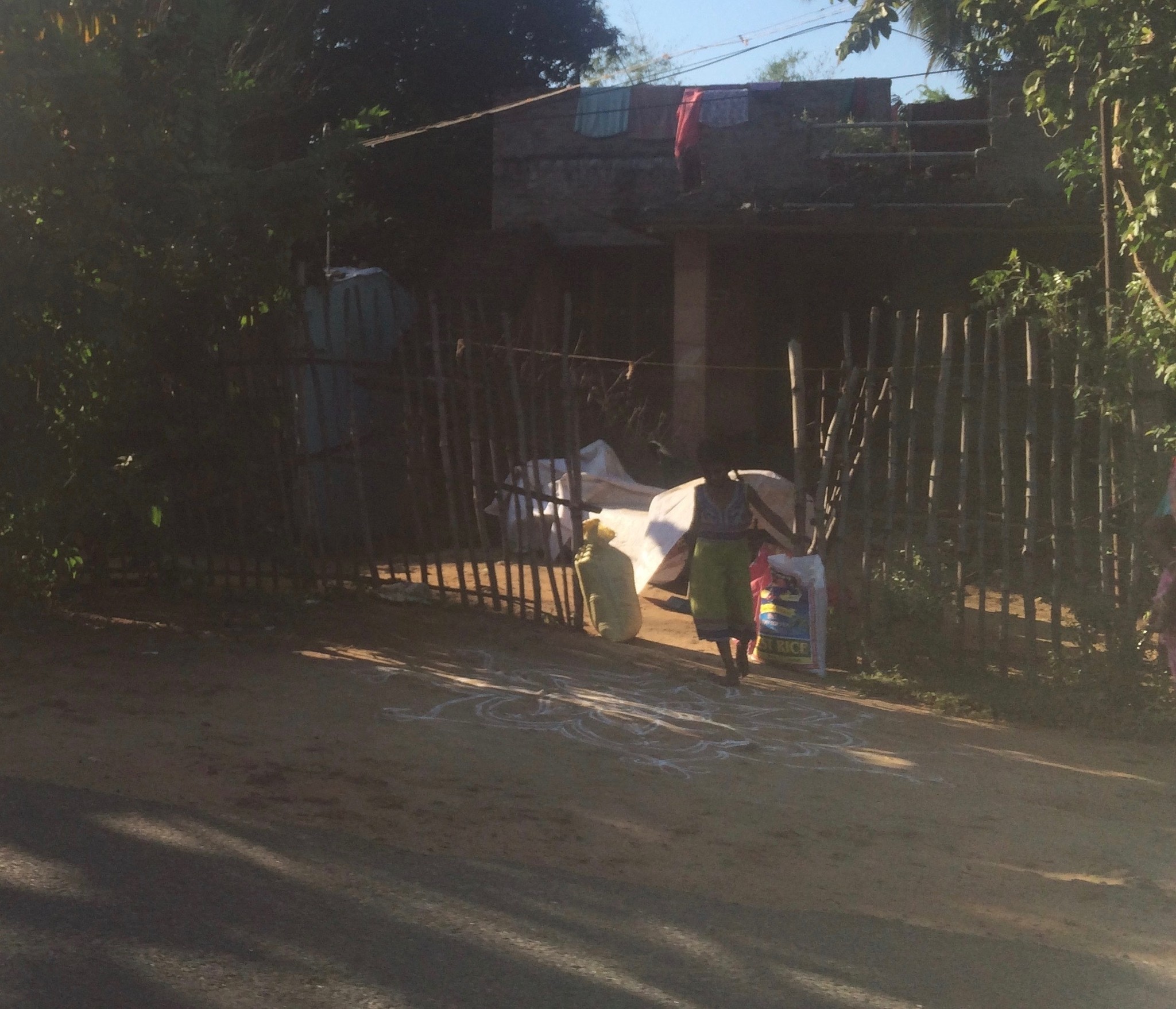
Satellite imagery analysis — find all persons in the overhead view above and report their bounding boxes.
[1141,514,1176,682]
[683,438,812,685]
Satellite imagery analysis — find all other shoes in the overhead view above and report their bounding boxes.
[735,641,749,677]
[719,675,741,686]
